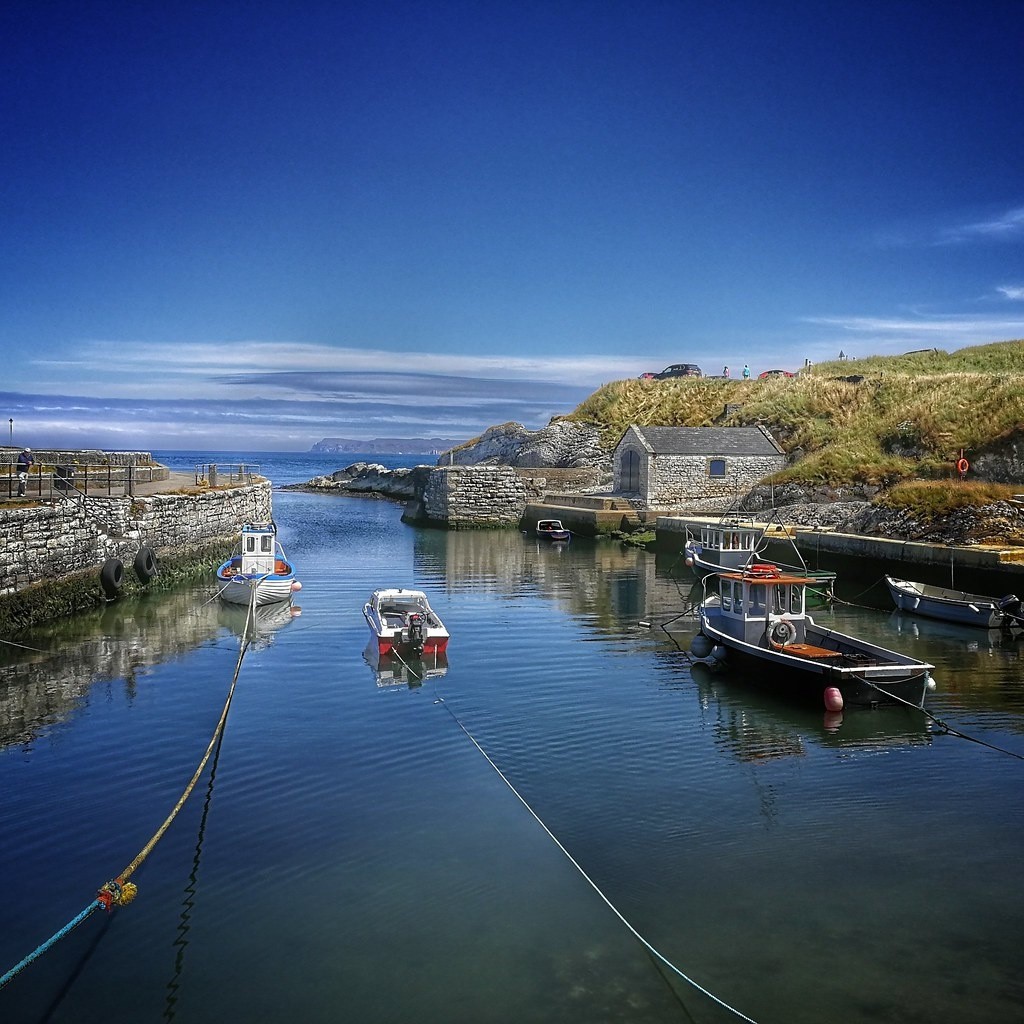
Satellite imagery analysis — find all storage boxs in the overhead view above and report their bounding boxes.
[845,652,876,668]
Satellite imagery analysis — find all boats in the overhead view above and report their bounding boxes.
[697,568,936,706]
[684,521,836,607]
[536,519,570,540]
[362,588,450,655]
[884,574,1021,628]
[216,524,296,607]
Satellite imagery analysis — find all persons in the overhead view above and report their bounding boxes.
[16,447,35,497]
[730,533,739,550]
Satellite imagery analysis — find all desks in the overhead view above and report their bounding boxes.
[777,643,843,667]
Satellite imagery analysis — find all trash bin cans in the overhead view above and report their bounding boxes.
[54,464,78,490]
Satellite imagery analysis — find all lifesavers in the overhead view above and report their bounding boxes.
[958,458,969,473]
[763,618,799,652]
[745,562,782,581]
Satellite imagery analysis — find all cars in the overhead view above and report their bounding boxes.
[759,370,794,380]
[639,373,656,379]
[652,364,701,380]
